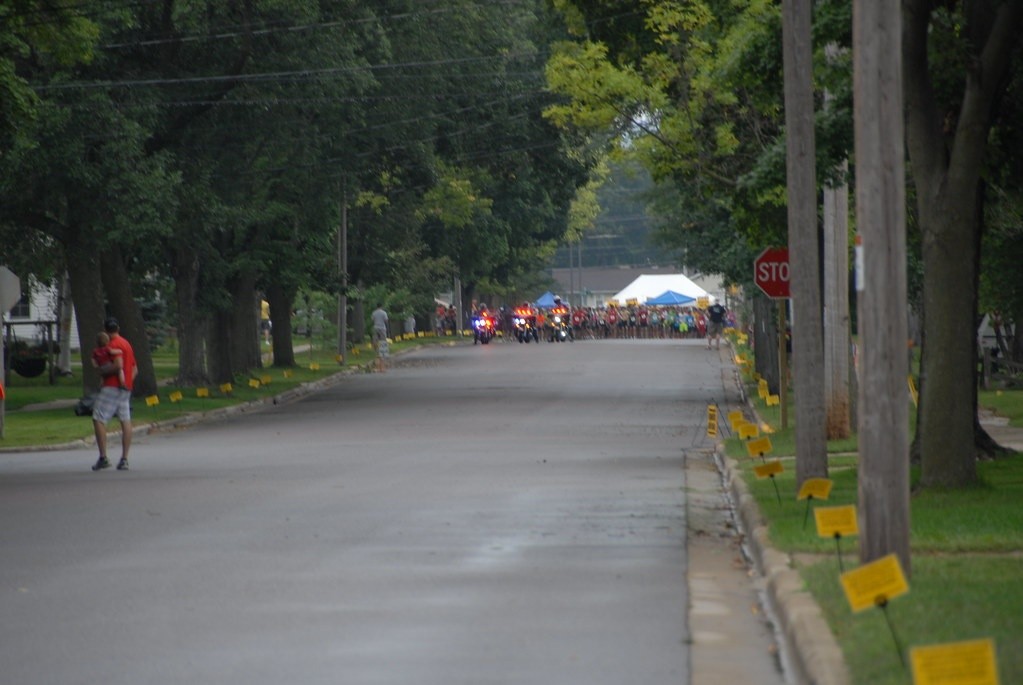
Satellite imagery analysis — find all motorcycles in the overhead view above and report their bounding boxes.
[471,308,498,344]
[512,308,538,343]
[546,308,574,342]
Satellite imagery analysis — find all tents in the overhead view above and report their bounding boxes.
[535,292,557,305]
[646,291,696,306]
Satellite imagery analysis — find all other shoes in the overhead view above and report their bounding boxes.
[119,384,129,391]
[92,455,111,471]
[97,388,101,392]
[117,458,129,470]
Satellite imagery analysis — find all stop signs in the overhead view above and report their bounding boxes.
[754,246,793,300]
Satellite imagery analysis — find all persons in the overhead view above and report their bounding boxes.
[371,303,389,357]
[472,295,734,343]
[704,298,726,349]
[435,304,457,336]
[261,295,271,344]
[91,317,138,470]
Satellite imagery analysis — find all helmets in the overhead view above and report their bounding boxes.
[553,295,561,303]
[480,302,486,308]
[524,302,529,307]
